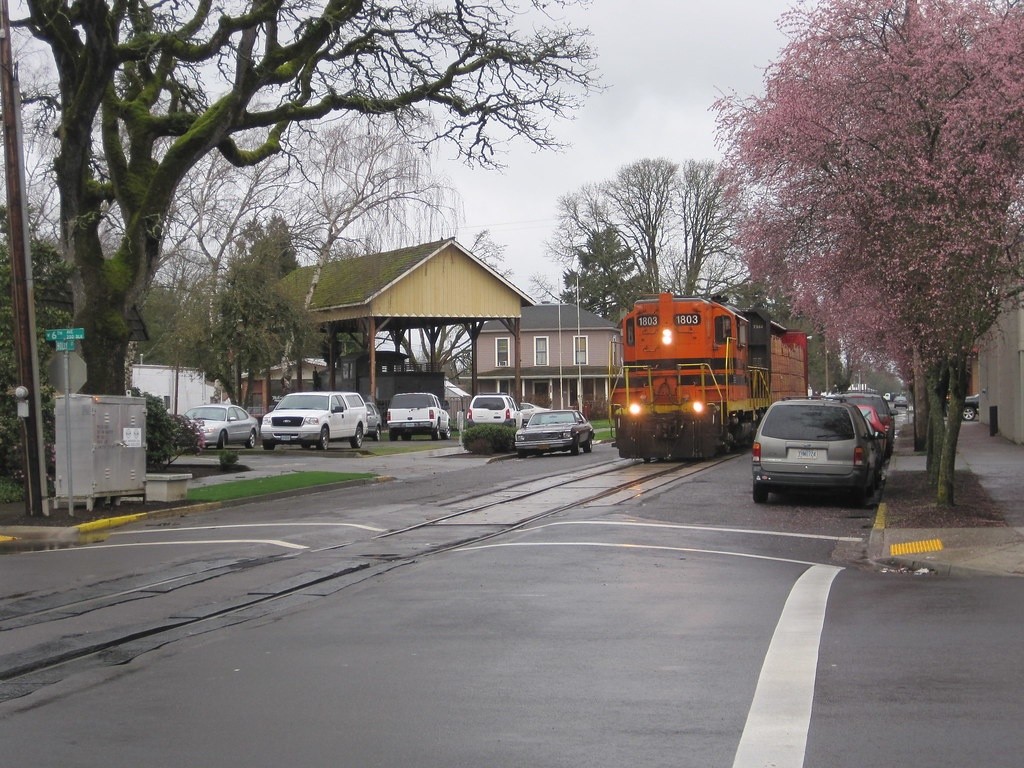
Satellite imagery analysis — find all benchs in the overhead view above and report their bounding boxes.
[146,472,193,503]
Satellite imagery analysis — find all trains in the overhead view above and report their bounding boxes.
[604,289,808,463]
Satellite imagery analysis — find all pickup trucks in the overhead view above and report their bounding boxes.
[261,392,367,449]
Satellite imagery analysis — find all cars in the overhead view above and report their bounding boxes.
[520,402,551,424]
[856,405,891,464]
[515,409,595,458]
[365,403,382,441]
[884,391,908,408]
[181,403,260,449]
[963,394,981,422]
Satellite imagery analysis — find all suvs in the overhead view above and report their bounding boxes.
[387,393,452,441]
[831,392,898,449]
[752,393,885,506]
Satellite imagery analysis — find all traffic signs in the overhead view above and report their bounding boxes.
[44,328,85,341]
[55,340,76,351]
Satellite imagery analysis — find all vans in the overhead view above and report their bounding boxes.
[466,393,524,428]
